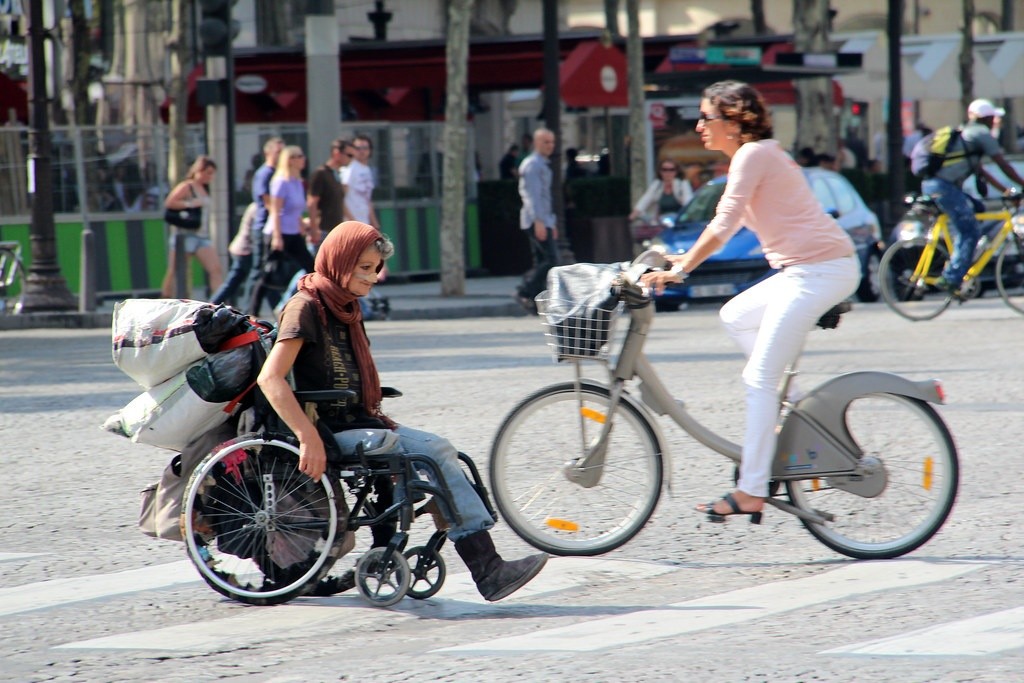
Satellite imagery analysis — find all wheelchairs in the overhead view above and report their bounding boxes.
[178,299,499,609]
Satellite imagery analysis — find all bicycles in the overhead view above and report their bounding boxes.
[487,253,961,562]
[877,183,1024,324]
[0,240,27,314]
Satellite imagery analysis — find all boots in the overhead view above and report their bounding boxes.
[453,528,548,601]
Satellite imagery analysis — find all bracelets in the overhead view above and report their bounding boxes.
[671,264,688,280]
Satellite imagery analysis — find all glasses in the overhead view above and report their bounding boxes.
[698,112,723,123]
[661,168,675,171]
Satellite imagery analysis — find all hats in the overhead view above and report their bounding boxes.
[967,99,1005,119]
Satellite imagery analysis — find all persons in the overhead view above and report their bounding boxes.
[97,100,1024,314]
[640,80,862,527]
[255,220,549,602]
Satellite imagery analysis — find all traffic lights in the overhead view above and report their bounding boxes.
[838,99,869,144]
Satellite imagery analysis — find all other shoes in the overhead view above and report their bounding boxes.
[934,275,965,302]
[511,289,537,316]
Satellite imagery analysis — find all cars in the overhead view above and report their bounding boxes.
[640,166,887,314]
[880,151,1024,288]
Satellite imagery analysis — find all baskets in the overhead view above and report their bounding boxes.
[535,290,624,360]
[631,225,665,244]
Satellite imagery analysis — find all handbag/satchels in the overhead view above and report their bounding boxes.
[99,298,265,543]
[547,251,666,324]
[164,184,202,229]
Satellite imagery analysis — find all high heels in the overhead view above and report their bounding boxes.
[691,494,761,524]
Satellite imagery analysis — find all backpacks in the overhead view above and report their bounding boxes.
[910,126,960,178]
